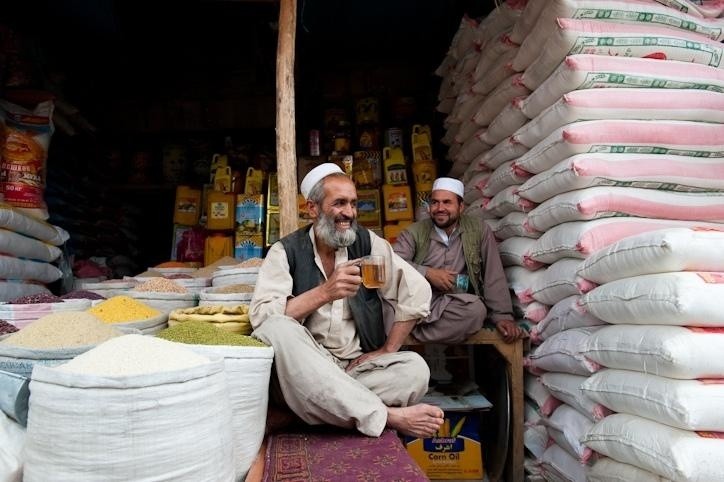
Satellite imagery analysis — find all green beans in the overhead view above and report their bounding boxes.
[6,292,64,304]
[0,319,20,336]
[61,290,106,299]
[153,320,270,347]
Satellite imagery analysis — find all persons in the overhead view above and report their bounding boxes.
[246,161,447,440]
[382,174,528,348]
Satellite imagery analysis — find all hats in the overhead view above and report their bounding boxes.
[432,178,464,199]
[300,162,345,202]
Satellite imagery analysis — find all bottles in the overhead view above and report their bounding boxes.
[231,170,241,194]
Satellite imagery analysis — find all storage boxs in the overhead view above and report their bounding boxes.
[404,412,483,481]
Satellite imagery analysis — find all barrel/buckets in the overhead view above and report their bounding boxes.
[170,97,438,266]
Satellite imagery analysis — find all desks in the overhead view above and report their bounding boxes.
[403,317,529,482]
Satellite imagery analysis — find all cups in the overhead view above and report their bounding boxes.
[352,255,387,288]
[451,274,470,294]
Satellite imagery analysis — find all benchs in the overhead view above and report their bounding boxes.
[241,420,433,482]
